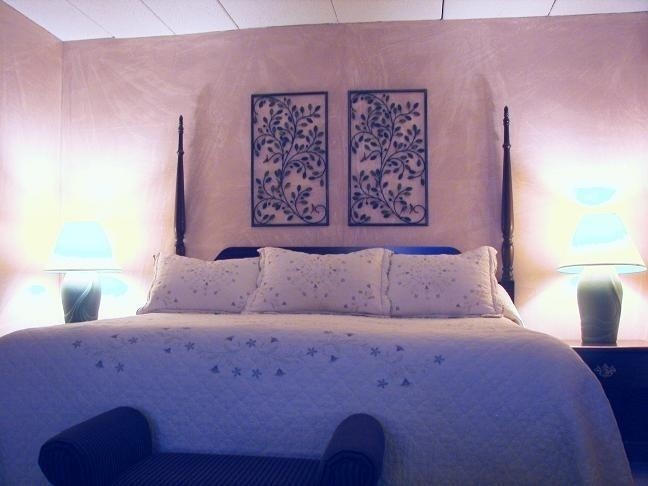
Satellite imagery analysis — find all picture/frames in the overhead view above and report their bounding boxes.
[250,89,429,227]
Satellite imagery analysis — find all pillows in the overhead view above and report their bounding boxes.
[384,248,503,319]
[242,247,392,320]
[135,248,262,315]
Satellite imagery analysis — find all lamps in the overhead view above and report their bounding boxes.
[43,221,122,324]
[555,213,648,346]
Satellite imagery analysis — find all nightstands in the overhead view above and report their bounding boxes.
[558,339,647,449]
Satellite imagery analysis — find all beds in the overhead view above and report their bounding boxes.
[0,106,635,486]
